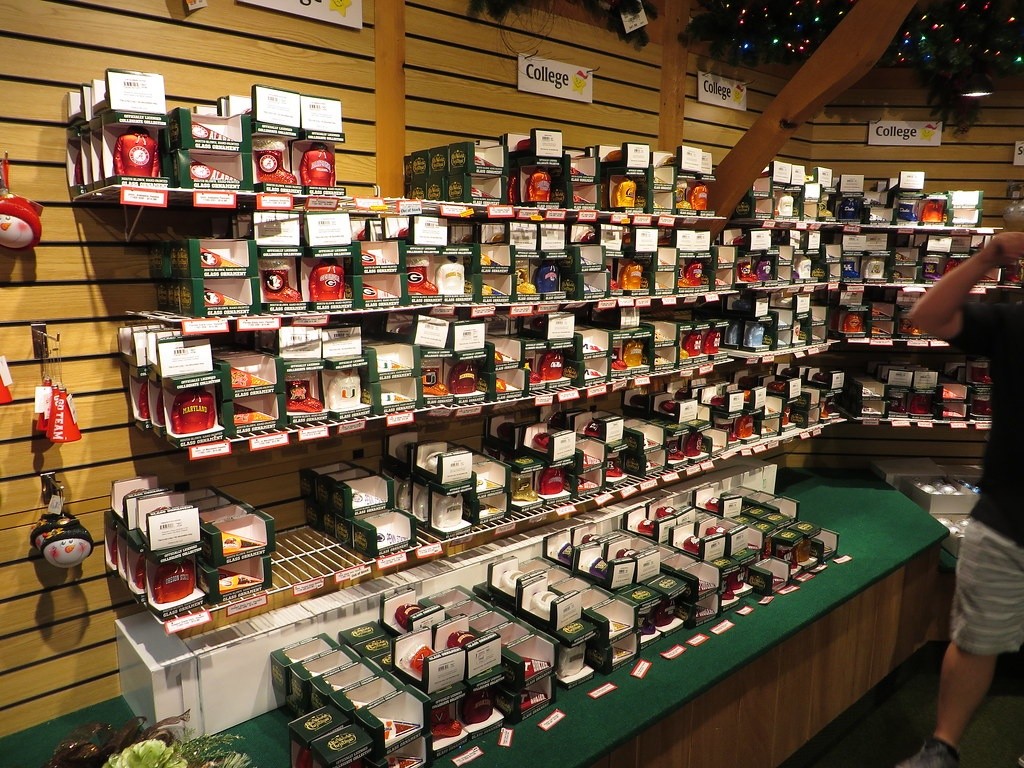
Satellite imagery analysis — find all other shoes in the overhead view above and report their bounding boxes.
[895,735,960,768]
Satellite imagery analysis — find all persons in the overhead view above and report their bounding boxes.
[897,232,1024,768]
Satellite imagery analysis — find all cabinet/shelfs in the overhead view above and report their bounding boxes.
[75,184,1024,634]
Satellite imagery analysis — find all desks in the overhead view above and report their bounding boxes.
[0,467,969,768]
